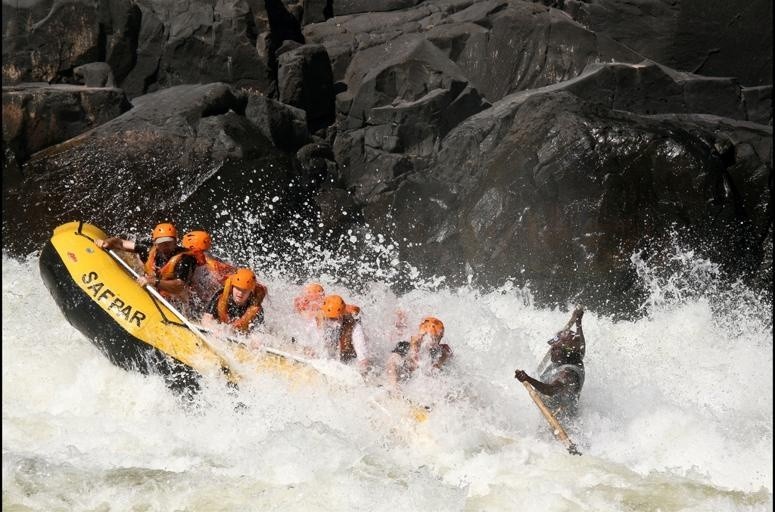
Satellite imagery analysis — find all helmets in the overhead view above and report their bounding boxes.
[181,230,212,251]
[230,268,257,291]
[418,316,445,336]
[547,329,587,361]
[321,294,346,318]
[304,283,325,301]
[345,303,361,314]
[151,223,177,242]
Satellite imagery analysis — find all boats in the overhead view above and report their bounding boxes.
[36,217,426,431]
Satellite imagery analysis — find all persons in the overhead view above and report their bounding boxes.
[293,283,373,376]
[96,224,267,333]
[386,318,453,400]
[514,311,586,443]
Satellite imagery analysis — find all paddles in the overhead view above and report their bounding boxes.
[110,250,242,374]
[194,322,365,388]
